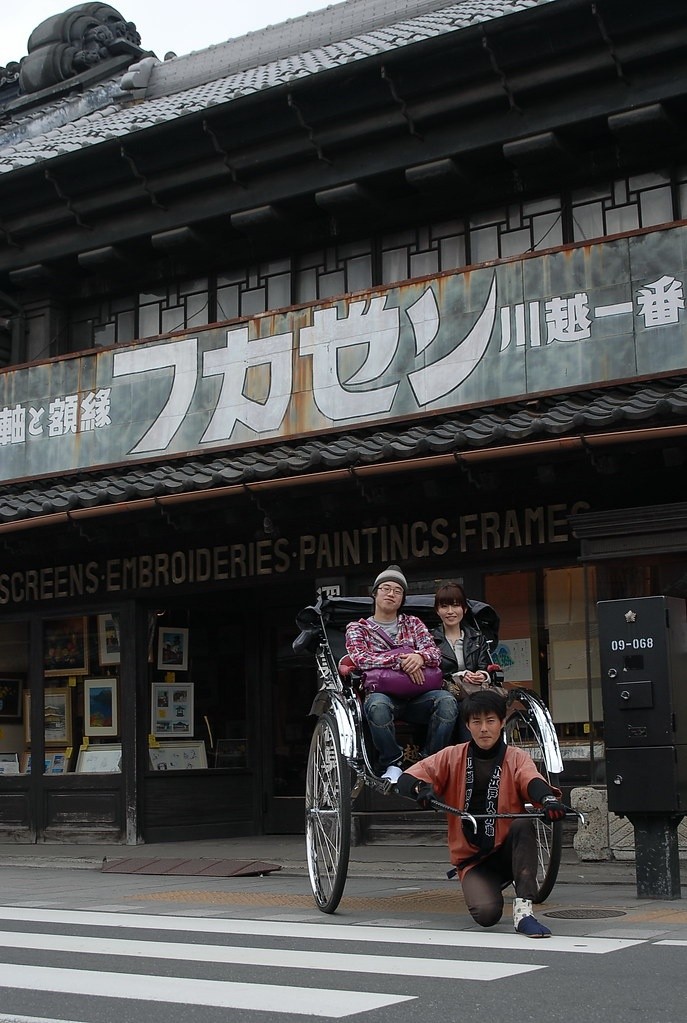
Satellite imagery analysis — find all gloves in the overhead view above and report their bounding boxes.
[543,796,566,824]
[415,783,440,811]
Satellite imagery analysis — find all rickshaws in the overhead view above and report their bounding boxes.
[292,589,584,913]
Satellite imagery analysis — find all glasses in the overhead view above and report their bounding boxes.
[376,587,404,596]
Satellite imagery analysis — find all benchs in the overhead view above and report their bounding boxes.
[315,596,511,722]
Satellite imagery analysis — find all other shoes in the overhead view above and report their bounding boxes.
[513,915,552,938]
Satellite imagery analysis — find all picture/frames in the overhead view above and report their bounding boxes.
[152,681,193,738]
[157,626,189,670]
[22,687,75,748]
[0,672,28,724]
[19,750,71,774]
[76,743,122,775]
[96,614,154,667]
[41,612,89,677]
[147,741,209,770]
[83,676,120,737]
[215,739,248,768]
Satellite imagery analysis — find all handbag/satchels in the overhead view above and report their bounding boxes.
[360,623,443,693]
[446,674,508,699]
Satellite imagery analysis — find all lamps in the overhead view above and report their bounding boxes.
[263,512,275,534]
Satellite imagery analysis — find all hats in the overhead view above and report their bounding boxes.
[371,565,407,593]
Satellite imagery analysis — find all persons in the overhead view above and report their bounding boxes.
[345,565,460,783]
[397,692,566,938]
[427,581,506,743]
